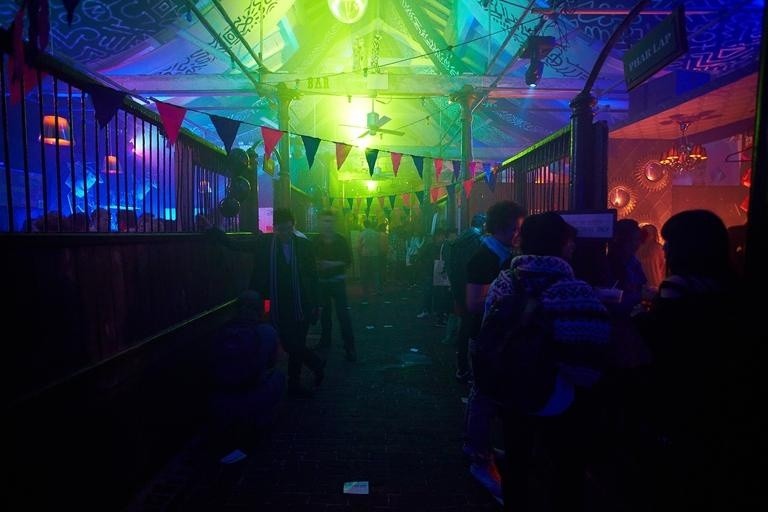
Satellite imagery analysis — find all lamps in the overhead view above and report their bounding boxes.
[658,122,707,176]
[100,156,123,174]
[37,114,75,147]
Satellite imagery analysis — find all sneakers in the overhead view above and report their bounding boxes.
[344,344,355,361]
[417,311,448,327]
[318,338,331,350]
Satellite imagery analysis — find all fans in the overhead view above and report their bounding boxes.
[339,98,406,140]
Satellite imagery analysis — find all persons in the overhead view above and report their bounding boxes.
[358,200,748,511]
[198,207,357,402]
[31,208,177,233]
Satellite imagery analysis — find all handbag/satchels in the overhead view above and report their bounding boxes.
[433,241,451,286]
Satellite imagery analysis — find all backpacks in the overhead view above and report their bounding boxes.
[541,281,608,372]
